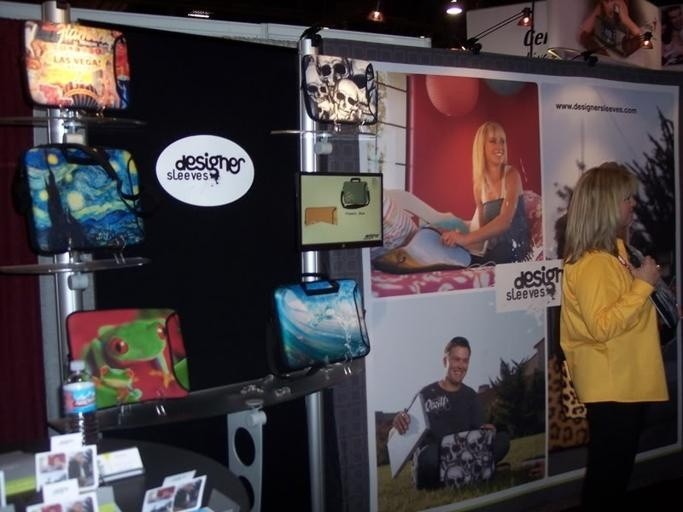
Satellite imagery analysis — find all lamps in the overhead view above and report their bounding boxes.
[446,0,463,14]
[464,7,530,47]
[570,31,652,61]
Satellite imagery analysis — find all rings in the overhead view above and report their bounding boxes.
[655,264,660,270]
[400,412,406,416]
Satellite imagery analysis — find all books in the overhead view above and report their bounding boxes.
[626,252,675,331]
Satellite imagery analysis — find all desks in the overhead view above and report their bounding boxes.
[0,436,250,512]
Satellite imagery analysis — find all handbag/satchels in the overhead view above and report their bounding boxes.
[66,307,190,411]
[562,358,588,419]
[270,272,370,370]
[341,177,370,209]
[441,428,497,489]
[301,55,379,126]
[21,18,130,112]
[20,140,148,255]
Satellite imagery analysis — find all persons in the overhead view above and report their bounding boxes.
[575,0,642,52]
[438,122,530,265]
[554,163,672,511]
[40,450,198,512]
[393,336,512,492]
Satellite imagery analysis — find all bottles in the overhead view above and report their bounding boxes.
[62,360,98,446]
